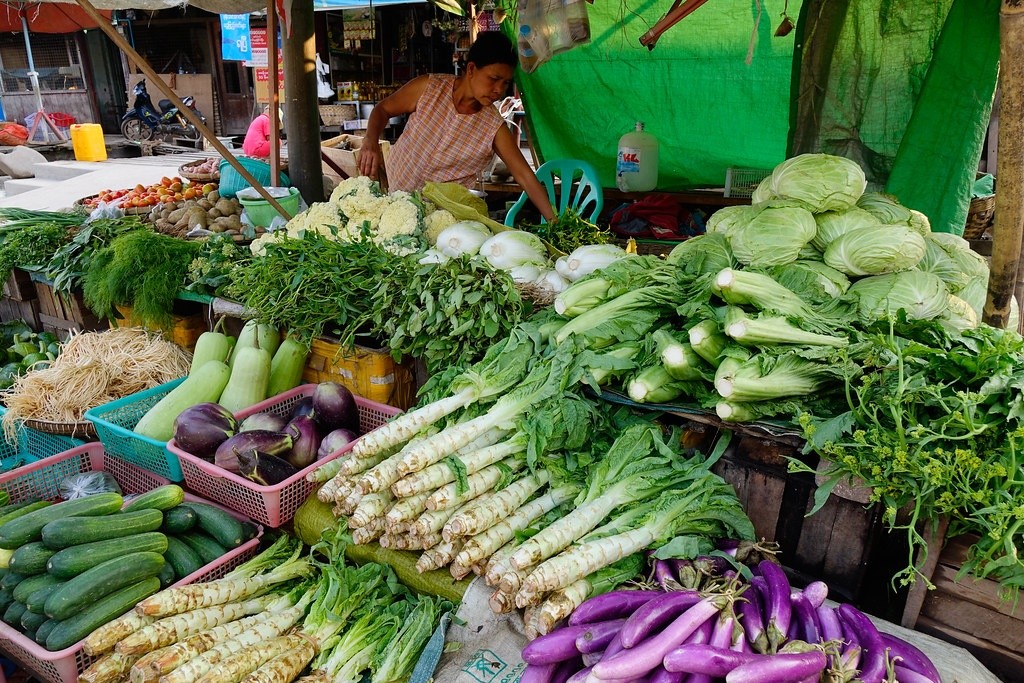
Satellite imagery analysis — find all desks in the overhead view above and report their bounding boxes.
[471,173,756,213]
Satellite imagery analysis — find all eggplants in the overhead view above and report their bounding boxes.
[520,544,944,683]
[172,380,362,504]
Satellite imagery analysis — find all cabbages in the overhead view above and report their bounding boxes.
[668,153,991,339]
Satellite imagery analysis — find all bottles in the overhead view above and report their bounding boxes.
[616,122,658,191]
[353,81,401,101]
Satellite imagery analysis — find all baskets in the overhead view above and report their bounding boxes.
[0,372,408,683]
[219,158,293,199]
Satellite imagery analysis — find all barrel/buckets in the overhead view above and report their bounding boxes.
[70,123,107,162]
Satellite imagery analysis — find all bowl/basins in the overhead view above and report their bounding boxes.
[238,187,300,228]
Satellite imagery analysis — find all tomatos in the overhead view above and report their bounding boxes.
[82,176,217,207]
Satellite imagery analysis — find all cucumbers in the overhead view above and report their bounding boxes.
[1,480,257,655]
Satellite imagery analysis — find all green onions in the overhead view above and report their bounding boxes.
[0,206,88,234]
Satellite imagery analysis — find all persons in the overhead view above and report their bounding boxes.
[241,104,284,165]
[350,29,558,228]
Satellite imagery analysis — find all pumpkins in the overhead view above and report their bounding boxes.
[132,315,308,461]
[1,330,63,391]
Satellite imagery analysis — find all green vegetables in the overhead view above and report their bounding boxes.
[1,222,240,341]
[76,540,453,682]
[227,221,1023,592]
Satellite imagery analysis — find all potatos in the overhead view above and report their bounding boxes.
[148,191,265,235]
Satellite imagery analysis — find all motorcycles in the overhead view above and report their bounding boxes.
[121,78,207,145]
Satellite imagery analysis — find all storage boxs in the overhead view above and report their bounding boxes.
[25,124,72,143]
[344,118,391,131]
[105,297,207,355]
[48,112,77,127]
[321,133,390,177]
[24,112,42,126]
[277,323,417,412]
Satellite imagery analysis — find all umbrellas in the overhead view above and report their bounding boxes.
[0,0,112,140]
